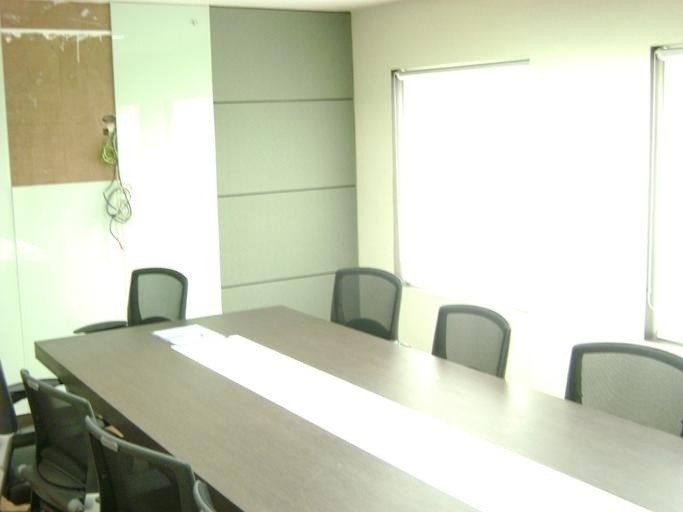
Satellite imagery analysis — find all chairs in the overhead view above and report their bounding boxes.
[73,268,187,335]
[433,304,510,377]
[331,269,401,340]
[566,343,683,437]
[0,359,217,512]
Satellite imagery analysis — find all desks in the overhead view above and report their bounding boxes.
[34,305,683,512]
